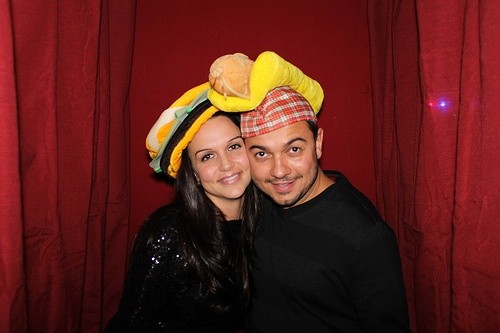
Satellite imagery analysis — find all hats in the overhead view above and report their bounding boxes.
[145,82,219,178]
[206,51,324,138]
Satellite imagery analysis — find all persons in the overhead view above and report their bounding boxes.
[241,84,411,333]
[107,81,252,333]
